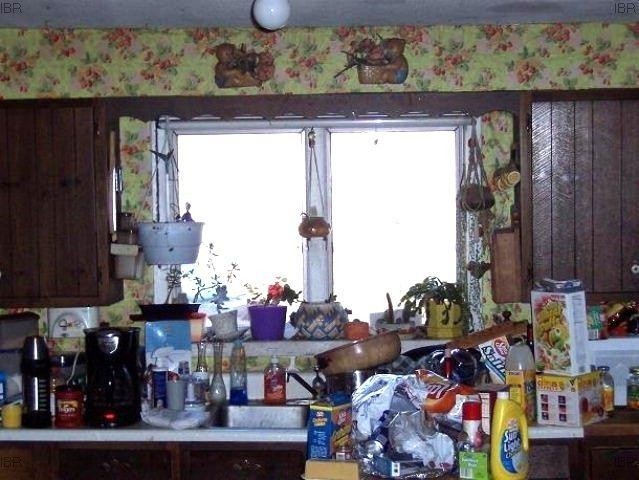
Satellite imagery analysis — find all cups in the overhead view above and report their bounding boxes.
[0,405,21,428]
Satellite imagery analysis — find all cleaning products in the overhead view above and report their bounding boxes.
[229,339,248,405]
[490,392,530,480]
[147,346,176,405]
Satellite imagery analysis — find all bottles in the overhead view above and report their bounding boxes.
[596,365,615,417]
[21,335,82,427]
[117,212,137,245]
[165,331,248,426]
[460,401,487,453]
[624,365,639,412]
[0,373,8,422]
[505,344,537,428]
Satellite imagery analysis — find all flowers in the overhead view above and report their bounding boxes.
[203,282,233,317]
[325,30,410,80]
[239,279,303,311]
[202,41,277,81]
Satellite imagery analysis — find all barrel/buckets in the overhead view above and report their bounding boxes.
[138,220,205,266]
[507,337,535,422]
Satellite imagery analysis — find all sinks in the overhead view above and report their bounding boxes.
[207,403,310,430]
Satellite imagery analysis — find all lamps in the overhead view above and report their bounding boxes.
[250,0,291,32]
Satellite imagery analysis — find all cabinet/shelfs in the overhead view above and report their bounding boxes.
[177,439,311,480]
[493,88,639,308]
[583,424,638,480]
[45,438,185,480]
[518,440,586,480]
[1,95,127,312]
[0,440,52,480]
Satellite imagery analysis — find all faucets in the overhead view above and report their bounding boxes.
[286,372,318,399]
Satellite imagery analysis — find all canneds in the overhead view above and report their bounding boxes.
[626,366,639,410]
[366,427,389,458]
[54,385,84,428]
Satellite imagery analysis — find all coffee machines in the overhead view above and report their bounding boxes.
[81,320,140,430]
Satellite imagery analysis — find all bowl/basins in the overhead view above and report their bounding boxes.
[140,397,221,431]
[313,327,404,377]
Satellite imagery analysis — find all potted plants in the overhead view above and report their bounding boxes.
[399,278,473,340]
[298,127,330,243]
[375,293,416,340]
[345,319,369,340]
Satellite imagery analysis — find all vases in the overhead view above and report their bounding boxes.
[206,308,239,338]
[213,63,264,88]
[130,217,206,269]
[244,302,289,342]
[356,59,408,84]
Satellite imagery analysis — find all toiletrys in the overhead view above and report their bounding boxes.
[262,347,286,406]
